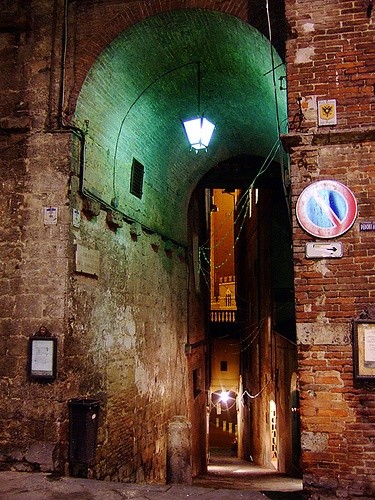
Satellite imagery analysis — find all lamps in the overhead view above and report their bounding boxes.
[178,109,216,154]
